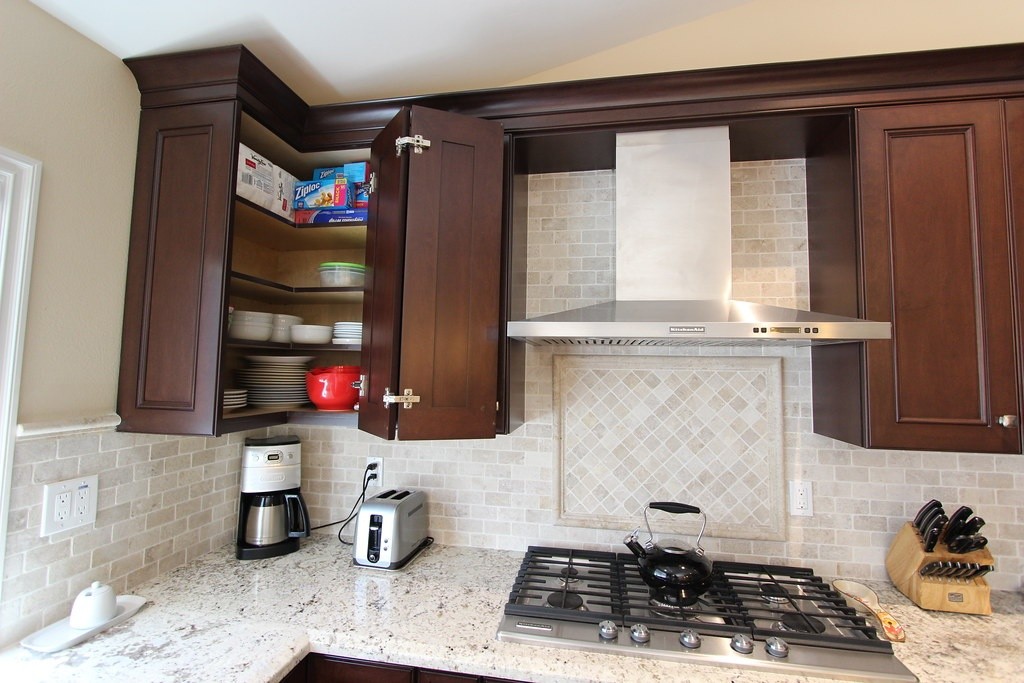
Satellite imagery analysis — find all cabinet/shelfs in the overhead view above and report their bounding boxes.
[797,41,1024,456]
[112,41,525,446]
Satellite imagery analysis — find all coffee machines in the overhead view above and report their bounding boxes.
[234,435,312,562]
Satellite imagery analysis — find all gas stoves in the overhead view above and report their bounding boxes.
[495,543,923,683]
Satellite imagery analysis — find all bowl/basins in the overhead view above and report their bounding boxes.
[318,262,365,288]
[305,364,363,411]
[227,305,333,346]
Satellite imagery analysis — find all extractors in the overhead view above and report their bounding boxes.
[506,126,898,358]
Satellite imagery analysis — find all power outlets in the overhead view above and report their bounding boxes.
[789,479,815,517]
[39,473,99,537]
[365,456,382,489]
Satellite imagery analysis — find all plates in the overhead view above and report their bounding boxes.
[17,592,149,654]
[331,321,363,345]
[220,352,315,414]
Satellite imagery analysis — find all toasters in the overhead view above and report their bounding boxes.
[353,487,427,571]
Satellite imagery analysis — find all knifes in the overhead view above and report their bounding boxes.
[912,498,996,584]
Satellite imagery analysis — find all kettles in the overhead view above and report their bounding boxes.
[625,501,715,604]
